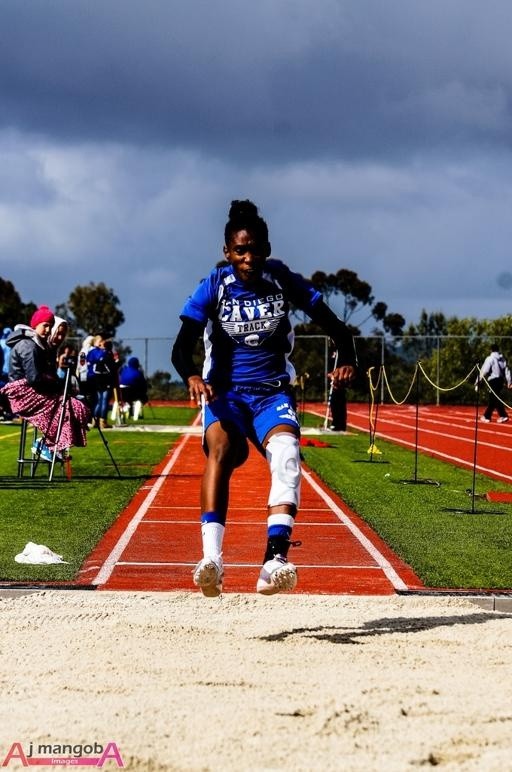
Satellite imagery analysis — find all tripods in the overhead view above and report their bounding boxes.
[31,368,121,482]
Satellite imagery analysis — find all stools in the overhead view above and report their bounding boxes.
[19,410,68,480]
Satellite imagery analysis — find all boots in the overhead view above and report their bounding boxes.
[100,418,112,428]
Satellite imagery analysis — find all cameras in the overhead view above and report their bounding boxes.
[63,356,78,368]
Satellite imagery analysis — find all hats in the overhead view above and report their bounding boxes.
[31,305,55,329]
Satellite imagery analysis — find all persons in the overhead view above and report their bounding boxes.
[37,314,91,433]
[326,346,348,432]
[8,306,88,462]
[170,197,361,596]
[473,344,511,424]
[0,327,150,430]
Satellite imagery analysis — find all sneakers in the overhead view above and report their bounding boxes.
[193,556,223,597]
[256,555,297,595]
[32,441,53,463]
